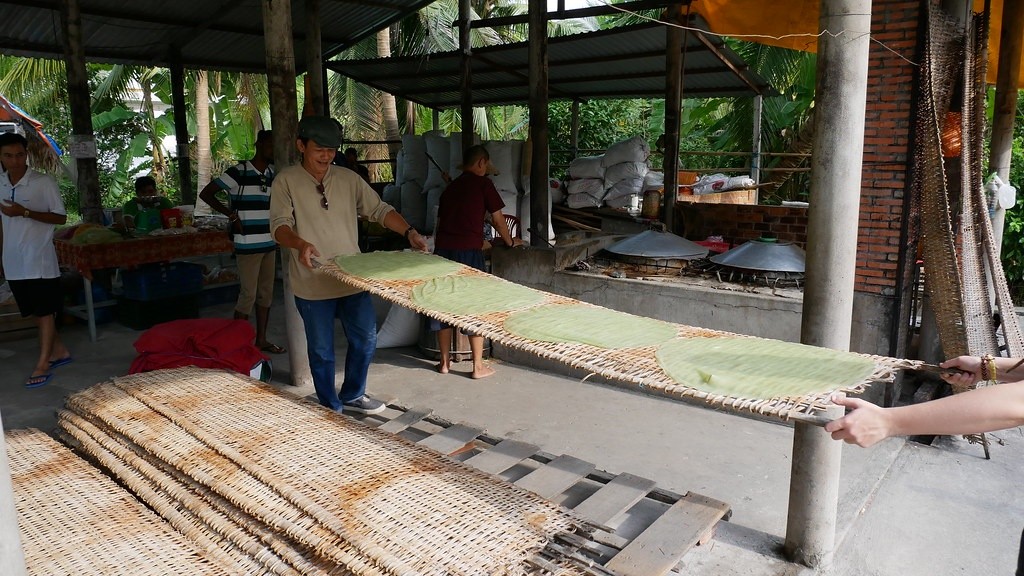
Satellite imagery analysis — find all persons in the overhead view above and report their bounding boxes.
[199,130,288,354]
[270,115,429,415]
[432,144,530,379]
[121,175,175,235]
[0,133,76,388]
[824,356,1024,576]
[331,118,345,166]
[340,148,371,253]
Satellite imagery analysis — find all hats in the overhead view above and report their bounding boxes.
[299,115,340,149]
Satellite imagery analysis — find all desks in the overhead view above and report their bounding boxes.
[55,232,234,342]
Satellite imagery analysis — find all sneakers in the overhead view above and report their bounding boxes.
[337,394,386,414]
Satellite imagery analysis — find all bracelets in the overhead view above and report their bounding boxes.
[229,212,238,223]
[509,238,515,248]
[404,226,414,239]
[987,354,996,380]
[980,355,989,381]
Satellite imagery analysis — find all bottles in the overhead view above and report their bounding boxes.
[984,180,998,220]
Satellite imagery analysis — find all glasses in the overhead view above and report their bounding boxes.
[259,174,267,193]
[316,185,328,210]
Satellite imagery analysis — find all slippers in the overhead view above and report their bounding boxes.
[25,373,54,388]
[47,358,73,369]
[255,343,287,354]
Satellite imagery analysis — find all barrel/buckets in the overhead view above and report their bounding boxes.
[161,207,181,229]
[643,189,661,218]
[180,204,194,226]
[103,207,122,227]
[223,359,273,386]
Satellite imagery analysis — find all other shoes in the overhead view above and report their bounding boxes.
[208,268,229,284]
[203,265,221,284]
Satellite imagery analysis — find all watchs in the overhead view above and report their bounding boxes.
[23,207,29,218]
